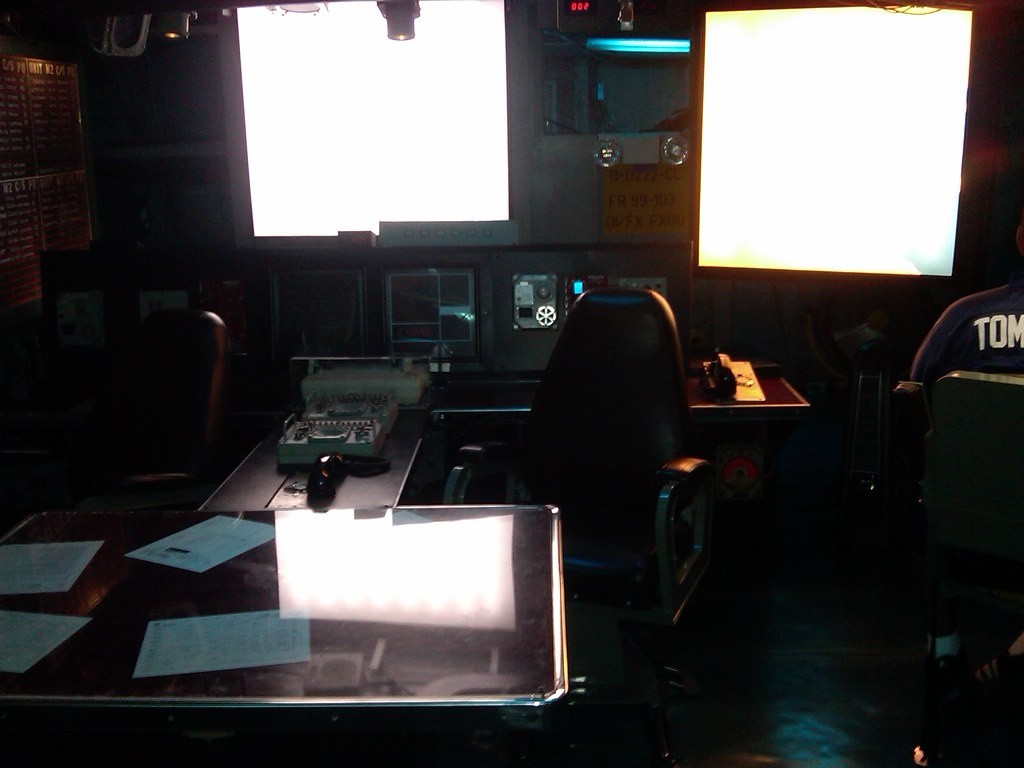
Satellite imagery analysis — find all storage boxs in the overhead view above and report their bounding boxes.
[298,361,427,405]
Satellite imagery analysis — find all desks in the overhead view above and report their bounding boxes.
[197,408,428,509]
[0,502,571,708]
[429,357,815,588]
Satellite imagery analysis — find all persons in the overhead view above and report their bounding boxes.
[910,210,1024,727]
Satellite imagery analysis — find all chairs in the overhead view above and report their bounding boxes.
[447,288,717,768]
[888,369,1024,768]
[235,264,377,439]
[0,304,234,536]
